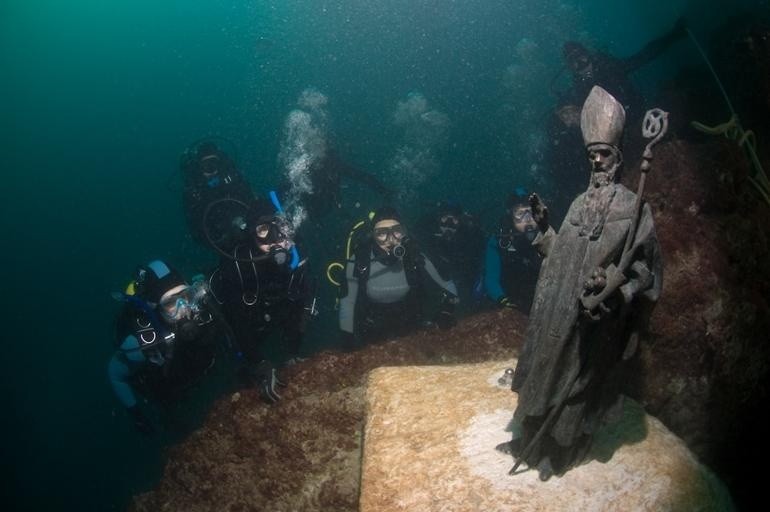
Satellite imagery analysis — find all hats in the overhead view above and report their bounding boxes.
[372,208,402,229]
[140,260,185,302]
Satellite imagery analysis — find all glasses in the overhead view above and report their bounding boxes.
[509,208,533,221]
[255,214,293,238]
[160,288,196,318]
[374,226,408,243]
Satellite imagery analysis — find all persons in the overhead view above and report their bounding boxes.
[280,129,394,213]
[331,208,456,349]
[488,194,546,305]
[497,116,661,476]
[563,22,688,111]
[212,208,315,403]
[176,140,254,220]
[102,259,219,432]
[540,101,596,185]
[421,202,492,307]
